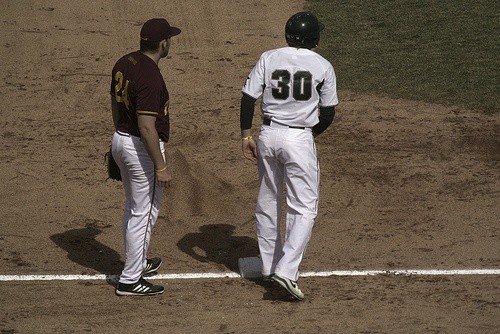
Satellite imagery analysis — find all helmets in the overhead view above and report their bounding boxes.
[285,11,325,48]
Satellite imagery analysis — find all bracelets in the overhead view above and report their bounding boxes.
[242,135,252,140]
[156,166,167,173]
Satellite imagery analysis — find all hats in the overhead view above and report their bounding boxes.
[140,17,181,41]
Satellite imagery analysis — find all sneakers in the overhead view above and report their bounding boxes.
[261,274,274,283]
[116,277,164,296]
[140,257,161,275]
[271,274,304,301]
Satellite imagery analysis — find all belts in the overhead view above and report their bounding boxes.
[263,117,305,130]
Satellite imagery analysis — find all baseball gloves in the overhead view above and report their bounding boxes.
[106,145,120,182]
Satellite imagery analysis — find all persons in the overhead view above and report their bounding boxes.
[239,12,338,301]
[110,18,181,295]
